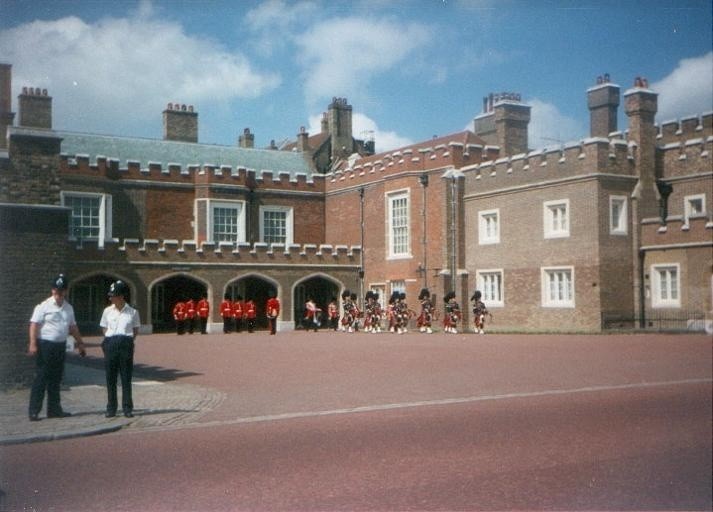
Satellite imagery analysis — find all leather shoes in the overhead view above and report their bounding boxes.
[105,411,133,418]
[47,412,71,417]
[29,413,37,420]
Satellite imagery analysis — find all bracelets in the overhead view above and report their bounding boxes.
[77,341,84,346]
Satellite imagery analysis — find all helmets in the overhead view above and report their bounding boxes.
[51,273,68,288]
[107,280,127,296]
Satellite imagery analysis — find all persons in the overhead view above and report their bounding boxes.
[342,290,411,334]
[443,292,460,333]
[266,290,279,334]
[99,280,141,418]
[327,298,338,331]
[27,276,86,421]
[172,293,209,335]
[219,293,256,334]
[305,295,321,333]
[471,291,487,334]
[417,290,434,334]
